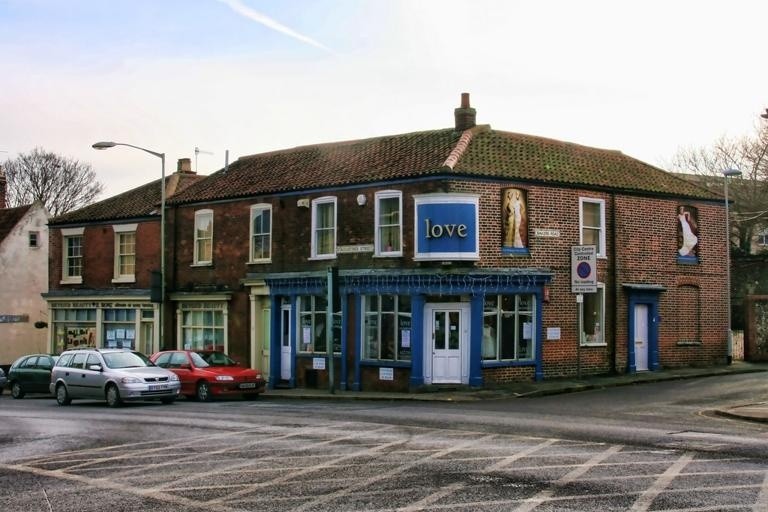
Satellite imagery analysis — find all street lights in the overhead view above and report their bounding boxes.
[723,168,742,365]
[91,141,166,351]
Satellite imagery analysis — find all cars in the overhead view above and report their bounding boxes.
[0,348,265,407]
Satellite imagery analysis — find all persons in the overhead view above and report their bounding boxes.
[505,190,514,246]
[513,190,525,249]
[677,206,697,258]
